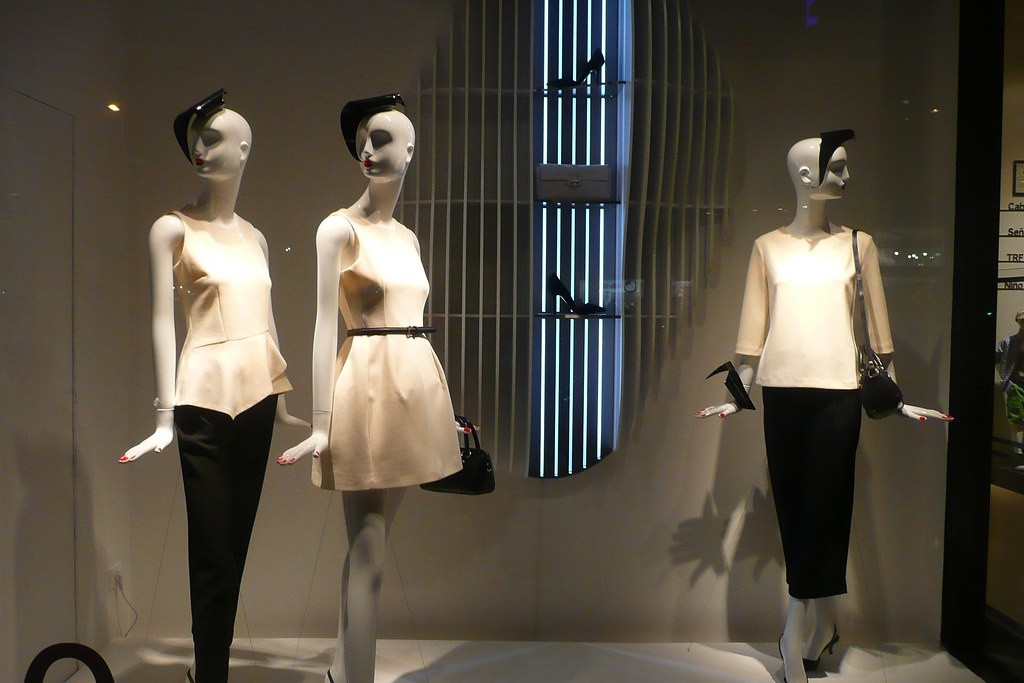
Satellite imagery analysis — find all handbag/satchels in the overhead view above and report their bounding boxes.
[860,345,902,420]
[536,162,611,202]
[420,413,496,496]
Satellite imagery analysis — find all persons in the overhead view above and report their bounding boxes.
[278,95,479,683]
[694,129,956,683]
[118,90,314,683]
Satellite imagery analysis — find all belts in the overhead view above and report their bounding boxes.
[345,326,437,337]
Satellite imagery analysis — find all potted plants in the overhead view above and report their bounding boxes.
[1005,379,1024,453]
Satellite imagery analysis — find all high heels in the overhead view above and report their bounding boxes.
[801,622,839,671]
[779,634,808,683]
[548,274,606,315]
[547,49,605,88]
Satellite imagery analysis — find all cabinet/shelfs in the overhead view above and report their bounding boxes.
[533,81,625,321]
